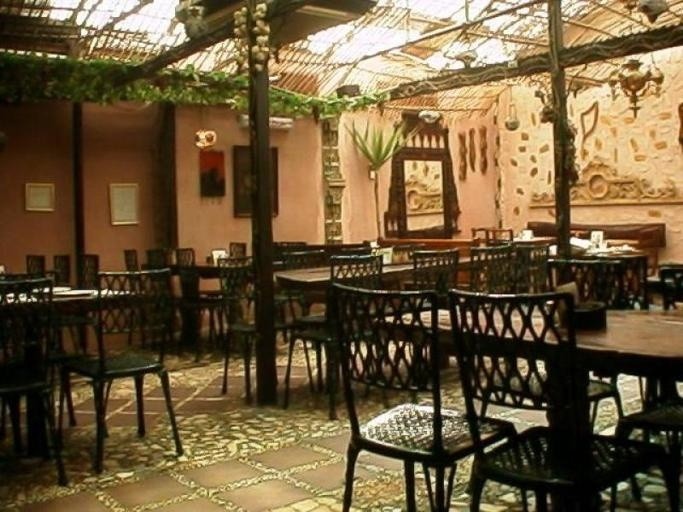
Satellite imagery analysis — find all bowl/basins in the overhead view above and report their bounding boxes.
[571,301,606,330]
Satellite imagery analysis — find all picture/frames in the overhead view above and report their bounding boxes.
[22,183,57,213]
[107,182,142,228]
[230,144,279,220]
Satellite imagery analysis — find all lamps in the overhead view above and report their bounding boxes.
[606,2,665,118]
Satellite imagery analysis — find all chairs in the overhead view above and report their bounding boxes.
[271,227,682,511]
[0,241,257,488]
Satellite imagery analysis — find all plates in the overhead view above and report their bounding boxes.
[30,287,93,297]
